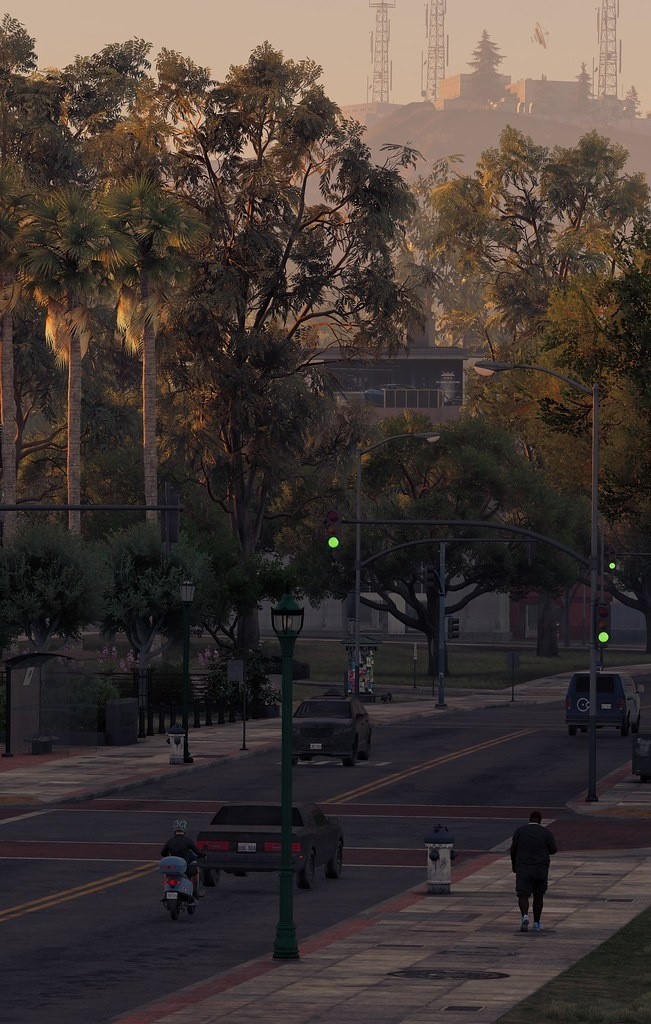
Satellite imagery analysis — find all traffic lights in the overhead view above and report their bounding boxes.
[596,605,610,644]
[452,618,459,638]
[608,547,616,571]
[326,509,340,551]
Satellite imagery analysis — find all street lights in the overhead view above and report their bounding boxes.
[354,432,439,695]
[271,580,305,960]
[474,360,599,805]
[179,579,195,763]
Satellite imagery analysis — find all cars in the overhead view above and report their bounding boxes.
[564,671,641,737]
[195,802,345,890]
[290,693,372,765]
[364,382,425,407]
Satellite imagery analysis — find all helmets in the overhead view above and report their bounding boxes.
[173,819,187,832]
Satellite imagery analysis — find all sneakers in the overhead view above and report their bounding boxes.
[520,914,529,932]
[532,921,543,931]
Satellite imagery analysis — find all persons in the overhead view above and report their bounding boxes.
[160,818,205,902]
[509,810,559,932]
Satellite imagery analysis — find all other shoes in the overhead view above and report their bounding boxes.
[159,896,164,901]
[194,890,204,898]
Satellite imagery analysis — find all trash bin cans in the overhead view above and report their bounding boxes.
[631,733,651,783]
[339,635,383,694]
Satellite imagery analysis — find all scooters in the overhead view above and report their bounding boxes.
[159,845,208,919]
